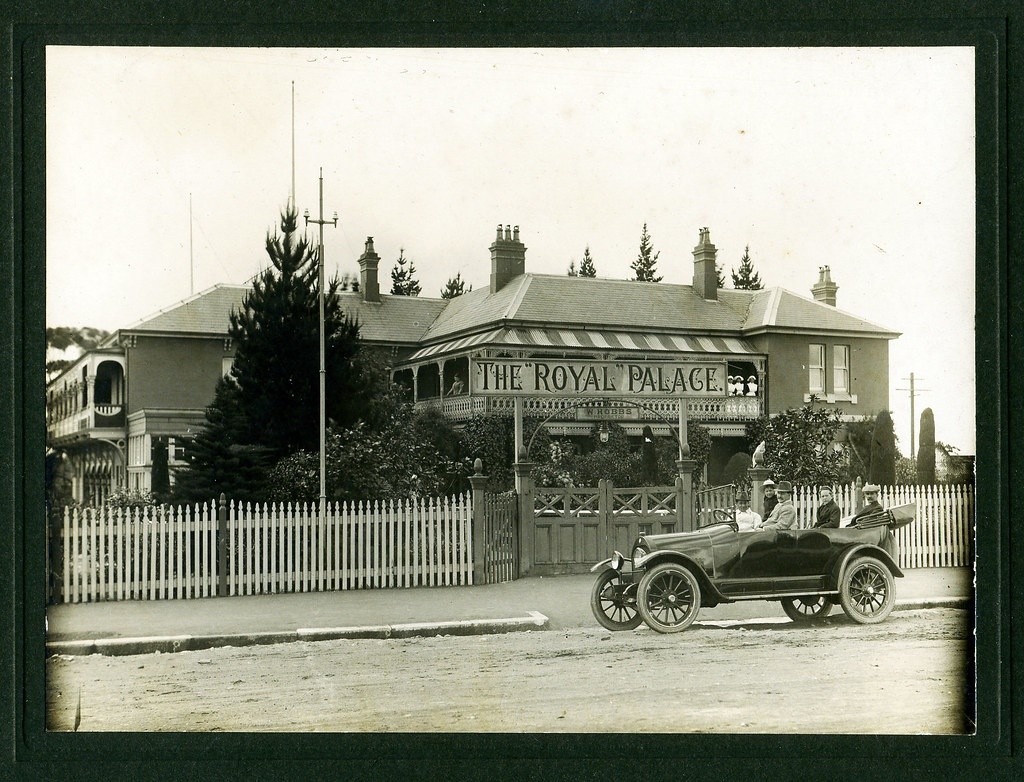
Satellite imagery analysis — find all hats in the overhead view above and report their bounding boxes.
[775,481,791,492]
[728,376,733,379]
[733,376,744,381]
[759,479,778,493]
[735,492,749,501]
[747,376,756,381]
[862,485,880,491]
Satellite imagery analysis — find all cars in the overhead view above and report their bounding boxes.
[590,502,918,634]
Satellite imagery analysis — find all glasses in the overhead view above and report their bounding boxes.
[728,379,732,380]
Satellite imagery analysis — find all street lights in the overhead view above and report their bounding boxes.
[304,168,338,558]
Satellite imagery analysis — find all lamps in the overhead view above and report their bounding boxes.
[598,401,610,442]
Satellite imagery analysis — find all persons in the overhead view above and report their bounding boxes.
[745,375,757,396]
[759,479,779,522]
[727,376,735,396]
[755,481,798,530]
[726,490,762,532]
[447,373,465,396]
[845,485,884,527]
[811,486,840,529]
[732,375,745,396]
